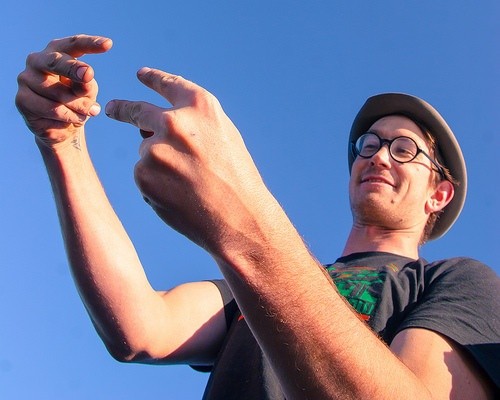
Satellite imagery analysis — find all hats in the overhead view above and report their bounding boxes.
[348,92,468,242]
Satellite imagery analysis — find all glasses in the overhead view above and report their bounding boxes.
[351,131,446,180]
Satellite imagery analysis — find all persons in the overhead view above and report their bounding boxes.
[15,34,500,400]
